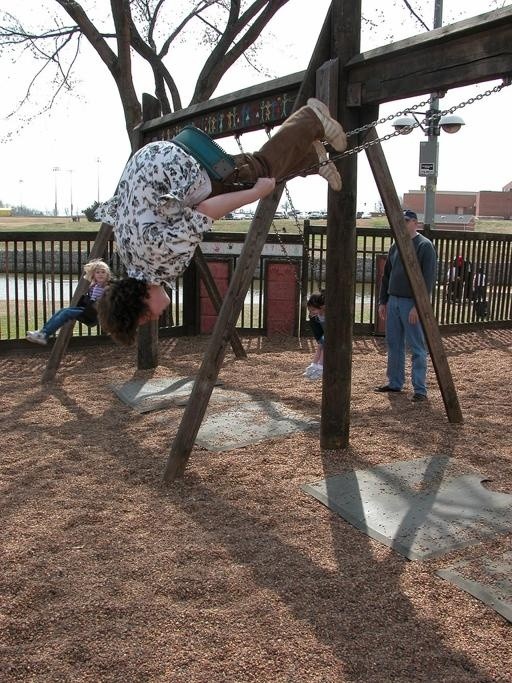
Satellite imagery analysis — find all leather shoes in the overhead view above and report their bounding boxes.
[411,393,428,402]
[374,385,403,392]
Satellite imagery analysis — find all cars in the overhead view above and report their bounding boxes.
[224,210,328,220]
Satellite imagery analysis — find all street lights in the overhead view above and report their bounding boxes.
[392,114,466,229]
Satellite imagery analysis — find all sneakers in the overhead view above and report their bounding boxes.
[303,363,326,380]
[311,140,343,193]
[307,97,347,152]
[26,330,47,345]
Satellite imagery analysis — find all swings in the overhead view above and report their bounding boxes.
[78,247,124,328]
[234,120,325,331]
[169,75,510,195]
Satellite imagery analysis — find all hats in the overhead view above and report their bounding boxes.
[403,209,418,220]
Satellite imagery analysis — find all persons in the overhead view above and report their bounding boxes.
[447,259,456,301]
[95,96,348,347]
[24,257,113,344]
[471,267,485,301]
[452,255,470,302]
[374,208,436,402]
[302,289,325,379]
